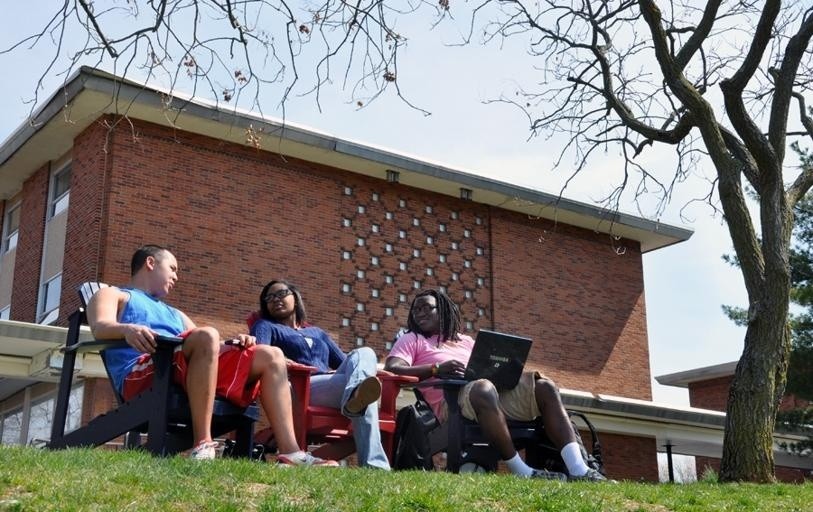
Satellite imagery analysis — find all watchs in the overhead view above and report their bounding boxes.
[435,362,440,373]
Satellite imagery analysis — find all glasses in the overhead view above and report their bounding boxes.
[411,305,437,313]
[263,289,293,302]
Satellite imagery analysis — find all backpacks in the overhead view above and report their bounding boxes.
[525,410,602,469]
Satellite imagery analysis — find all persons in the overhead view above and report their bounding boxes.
[388,287,610,481]
[251,277,394,470]
[85,245,340,467]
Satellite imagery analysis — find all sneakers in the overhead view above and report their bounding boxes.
[276,451,339,467]
[568,468,607,482]
[192,435,218,460]
[530,468,566,482]
[345,377,381,413]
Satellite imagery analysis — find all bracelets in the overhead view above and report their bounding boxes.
[432,360,436,374]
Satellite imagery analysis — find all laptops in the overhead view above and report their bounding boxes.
[433,329,533,388]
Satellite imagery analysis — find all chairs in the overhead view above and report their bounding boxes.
[393,373,603,478]
[246,310,418,472]
[47,279,264,460]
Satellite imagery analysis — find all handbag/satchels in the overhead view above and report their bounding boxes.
[399,401,447,461]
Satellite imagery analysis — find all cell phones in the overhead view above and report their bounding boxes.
[225,338,247,346]
